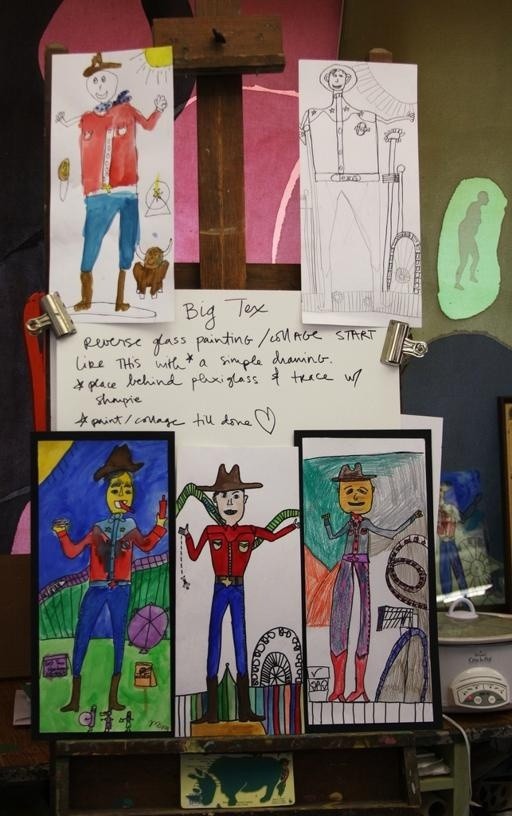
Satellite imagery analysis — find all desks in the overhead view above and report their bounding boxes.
[42,721,421,816]
[415,710,512,815]
